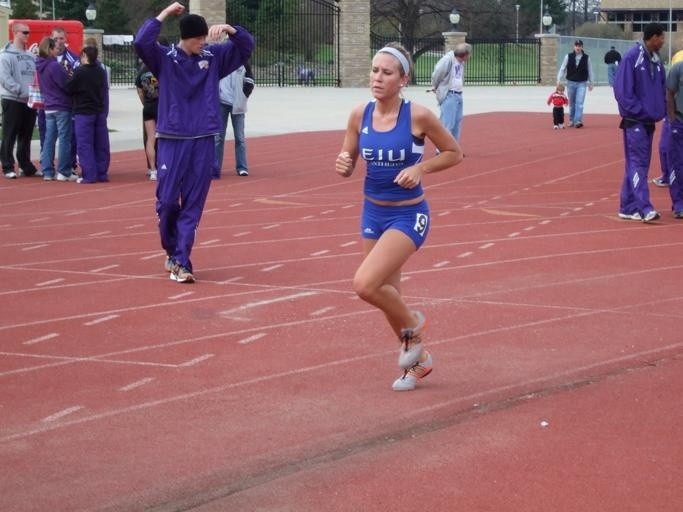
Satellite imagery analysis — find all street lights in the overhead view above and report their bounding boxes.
[514,4,520,42]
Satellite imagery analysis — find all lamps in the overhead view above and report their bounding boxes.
[85,3,99,28]
[449,8,461,32]
[542,7,554,34]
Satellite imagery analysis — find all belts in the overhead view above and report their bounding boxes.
[448,90,461,94]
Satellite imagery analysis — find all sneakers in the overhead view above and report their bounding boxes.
[165,257,195,283]
[618,207,683,223]
[391,351,432,392]
[652,178,667,186]
[3,169,83,184]
[552,121,583,130]
[237,171,248,175]
[397,311,428,368]
[146,167,157,181]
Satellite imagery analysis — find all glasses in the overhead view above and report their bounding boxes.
[52,36,64,40]
[47,38,53,49]
[15,31,29,35]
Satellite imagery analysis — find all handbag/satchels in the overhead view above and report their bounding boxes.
[27,82,43,109]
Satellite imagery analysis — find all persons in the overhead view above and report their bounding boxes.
[431,41,472,161]
[0,22,110,184]
[335,42,462,391]
[132,2,254,284]
[135,61,163,180]
[546,22,682,223]
[213,61,253,179]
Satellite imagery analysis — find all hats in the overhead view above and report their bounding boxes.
[180,14,208,38]
[574,40,582,45]
